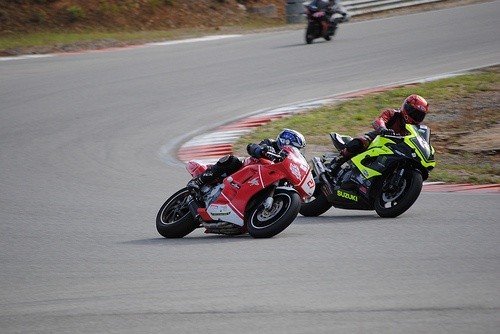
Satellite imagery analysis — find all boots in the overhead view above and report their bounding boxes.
[324,152,348,177]
[187,168,215,201]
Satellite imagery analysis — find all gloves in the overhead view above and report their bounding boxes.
[257,146,275,157]
[380,129,394,137]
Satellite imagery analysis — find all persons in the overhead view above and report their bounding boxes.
[328,94,429,179]
[307,0,333,42]
[187,129,306,235]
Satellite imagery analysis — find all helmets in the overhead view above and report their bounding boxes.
[401,94,428,126]
[276,128,306,151]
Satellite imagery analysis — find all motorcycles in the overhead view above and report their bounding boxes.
[298,5,347,45]
[155,145,316,240]
[298,121,437,218]
